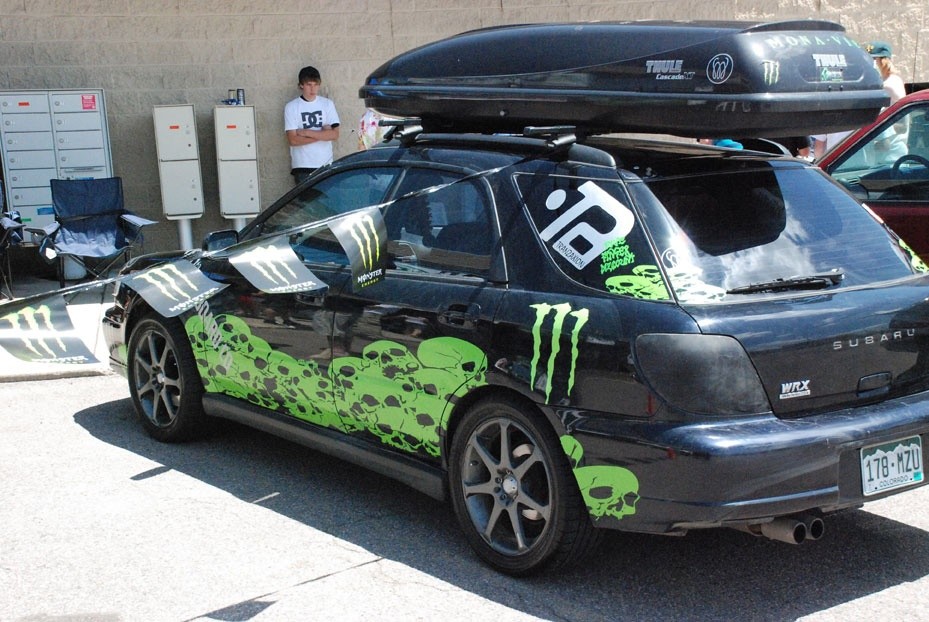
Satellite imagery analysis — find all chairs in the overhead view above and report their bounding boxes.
[21,176,159,305]
[0,181,24,300]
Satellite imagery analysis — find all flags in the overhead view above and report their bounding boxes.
[118,259,230,319]
[221,238,329,296]
[326,212,389,291]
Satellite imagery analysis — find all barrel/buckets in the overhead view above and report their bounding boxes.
[63,255,87,280]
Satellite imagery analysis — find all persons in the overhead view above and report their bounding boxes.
[282,65,342,188]
[705,45,910,171]
[357,101,424,252]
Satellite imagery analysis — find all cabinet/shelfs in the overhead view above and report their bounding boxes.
[0,88,113,240]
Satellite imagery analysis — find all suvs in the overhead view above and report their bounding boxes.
[105,19,928,582]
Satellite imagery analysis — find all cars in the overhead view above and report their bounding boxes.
[814,89,929,269]
